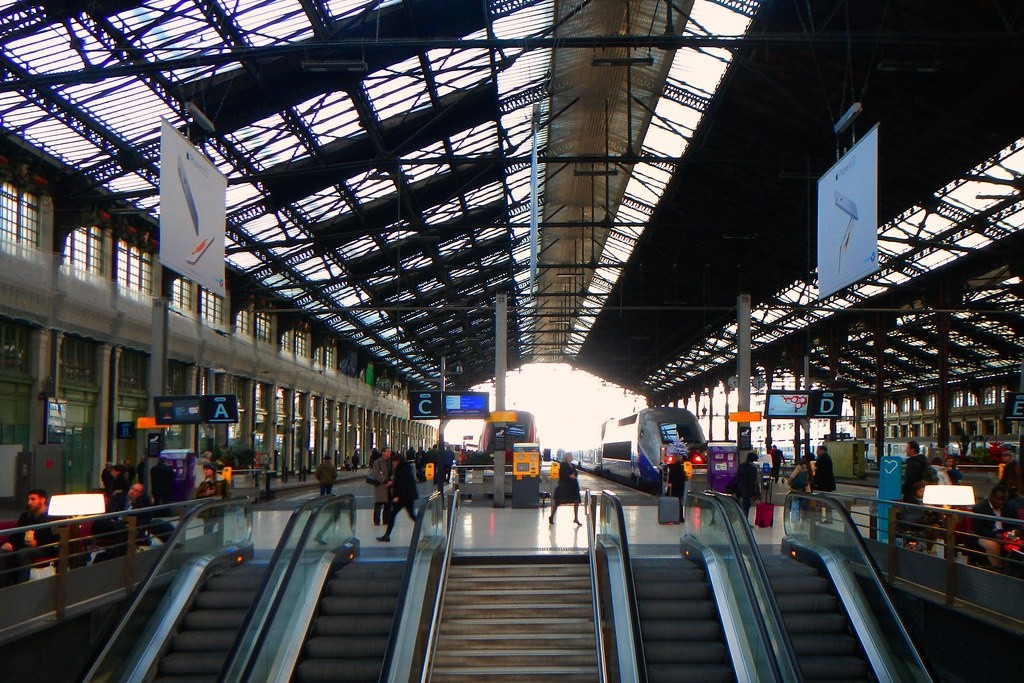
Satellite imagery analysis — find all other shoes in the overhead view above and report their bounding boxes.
[376,533,390,542]
[574,520,582,525]
[549,517,554,525]
[822,520,833,524]
[374,520,380,526]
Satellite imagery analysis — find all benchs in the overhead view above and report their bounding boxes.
[891,499,1024,574]
[0,513,107,581]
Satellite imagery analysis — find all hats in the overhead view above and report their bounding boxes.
[912,480,926,492]
[203,462,218,470]
[323,456,331,460]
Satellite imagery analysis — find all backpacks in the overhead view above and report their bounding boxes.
[916,458,939,486]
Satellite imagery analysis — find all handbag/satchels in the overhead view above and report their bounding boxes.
[365,472,385,485]
[790,465,808,489]
[726,477,740,493]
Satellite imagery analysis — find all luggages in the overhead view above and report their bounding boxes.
[657,485,680,525]
[754,477,774,528]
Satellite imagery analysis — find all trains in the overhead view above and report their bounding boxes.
[551,408,707,495]
[477,411,536,468]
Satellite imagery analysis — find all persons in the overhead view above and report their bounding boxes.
[192,451,211,489]
[315,456,338,496]
[101,462,131,492]
[735,452,759,528]
[666,455,685,522]
[375,453,419,542]
[770,444,785,483]
[0,489,53,588]
[372,447,393,525]
[787,446,836,524]
[314,505,337,544]
[971,461,1024,562]
[547,452,581,525]
[897,441,962,554]
[90,483,150,560]
[406,444,454,484]
[123,455,144,483]
[150,457,172,516]
[344,452,359,472]
[196,463,231,534]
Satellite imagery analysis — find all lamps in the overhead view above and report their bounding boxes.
[185,102,216,132]
[835,103,863,135]
[549,317,570,324]
[573,162,619,176]
[923,485,975,508]
[302,42,368,72]
[47,494,106,518]
[557,268,585,277]
[590,47,654,66]
